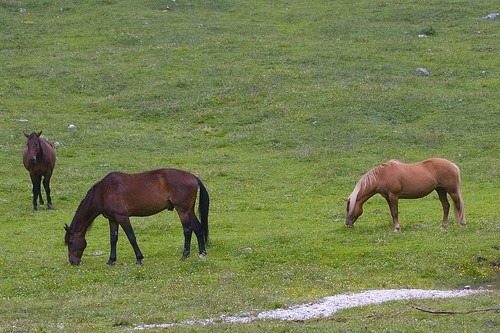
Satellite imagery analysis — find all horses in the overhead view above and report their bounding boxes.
[64,168,210,267]
[23,130,56,212]
[343,157,466,233]
